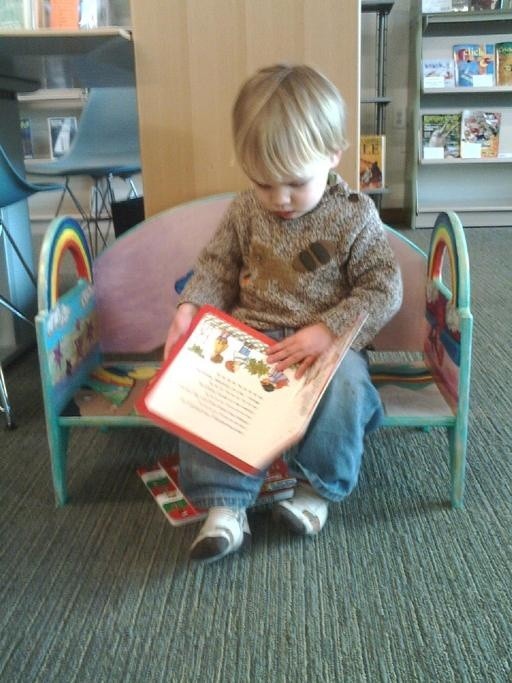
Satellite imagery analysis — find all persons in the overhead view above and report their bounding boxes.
[161,63,404,561]
[54,117,72,158]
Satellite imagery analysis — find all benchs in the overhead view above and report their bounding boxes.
[32,191,474,509]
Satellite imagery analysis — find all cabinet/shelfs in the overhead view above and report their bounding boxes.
[404,2,512,229]
[360,0,394,219]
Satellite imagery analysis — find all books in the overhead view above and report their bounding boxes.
[360,134,387,188]
[158,449,297,514]
[136,466,295,527]
[0,0,111,28]
[459,109,501,158]
[421,112,460,158]
[423,58,455,87]
[133,300,371,478]
[48,116,78,159]
[496,41,512,85]
[452,44,496,87]
